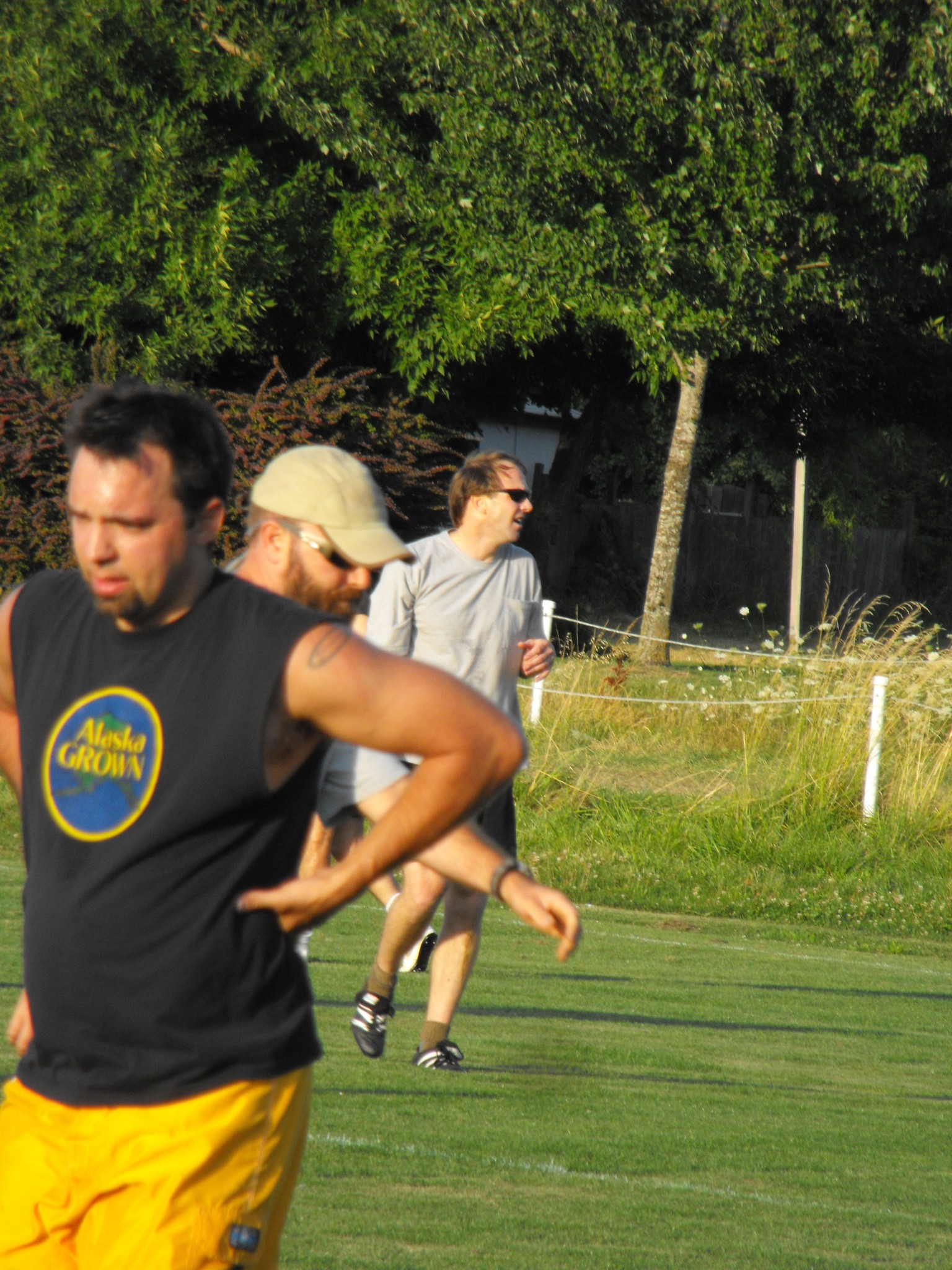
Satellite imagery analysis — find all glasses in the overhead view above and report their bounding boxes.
[486,486,529,504]
[247,517,354,569]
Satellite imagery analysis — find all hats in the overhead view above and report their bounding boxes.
[251,445,415,567]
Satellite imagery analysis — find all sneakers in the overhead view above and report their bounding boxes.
[294,932,312,965]
[397,927,437,976]
[351,979,397,1058]
[412,1039,463,1072]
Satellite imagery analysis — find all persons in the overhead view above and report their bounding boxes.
[0,381,531,1270]
[349,449,557,1073]
[293,805,438,976]
[9,449,583,1057]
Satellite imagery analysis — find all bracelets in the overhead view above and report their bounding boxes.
[491,857,532,903]
